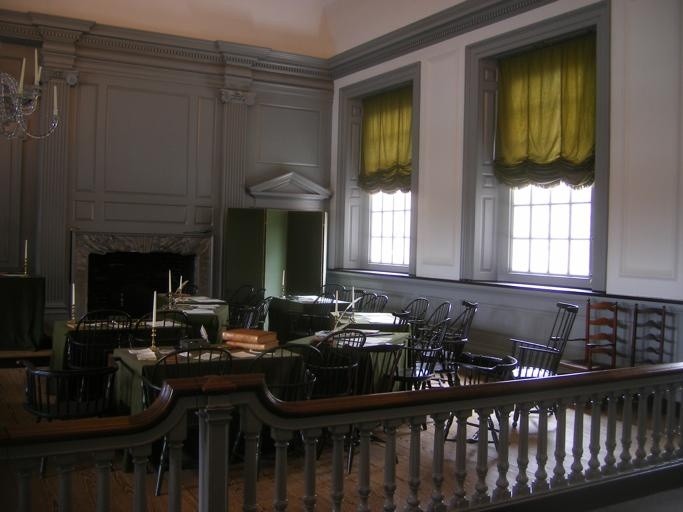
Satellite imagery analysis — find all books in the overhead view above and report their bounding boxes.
[222,328,279,351]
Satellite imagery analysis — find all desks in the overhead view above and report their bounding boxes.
[0,272,45,368]
[112,328,413,475]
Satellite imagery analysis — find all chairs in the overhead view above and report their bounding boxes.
[250,343,324,400]
[380,342,445,431]
[308,354,363,474]
[443,353,518,446]
[21,361,120,480]
[66,285,479,365]
[510,301,578,427]
[622,304,667,368]
[146,345,232,495]
[559,297,619,410]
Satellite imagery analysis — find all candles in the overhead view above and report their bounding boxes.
[30,48,43,89]
[23,239,28,276]
[17,56,26,97]
[172,275,189,298]
[279,270,285,298]
[167,269,172,310]
[50,84,60,118]
